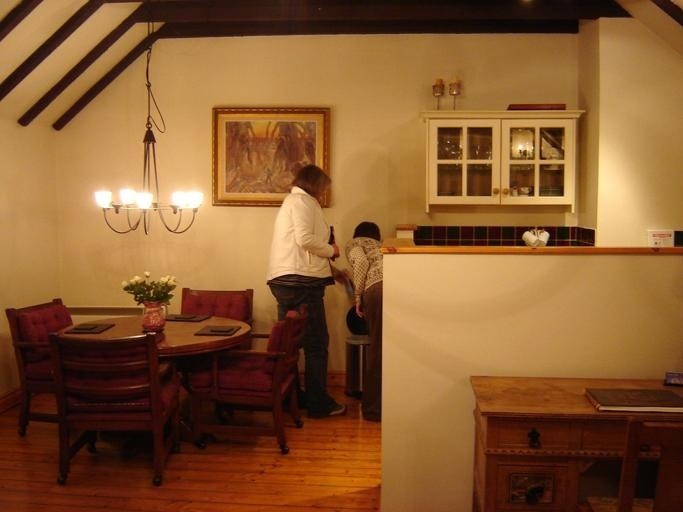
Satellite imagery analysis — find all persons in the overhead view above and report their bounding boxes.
[340,220,383,423]
[264,163,349,420]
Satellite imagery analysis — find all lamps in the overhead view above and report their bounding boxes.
[95,22,203,235]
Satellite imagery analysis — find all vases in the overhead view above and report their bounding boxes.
[140,300,165,333]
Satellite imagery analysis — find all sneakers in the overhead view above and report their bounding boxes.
[328,402,347,416]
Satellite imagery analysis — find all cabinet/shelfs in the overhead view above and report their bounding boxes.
[420,110,585,214]
[470,376,683,512]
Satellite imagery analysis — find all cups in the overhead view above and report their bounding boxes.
[523,231,550,247]
[439,135,491,159]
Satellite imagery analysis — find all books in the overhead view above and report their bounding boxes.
[584,386,683,412]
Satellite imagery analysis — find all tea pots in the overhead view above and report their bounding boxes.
[140,300,169,334]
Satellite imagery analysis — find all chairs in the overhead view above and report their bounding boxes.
[586,416,682,512]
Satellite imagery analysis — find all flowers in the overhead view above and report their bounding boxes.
[121,271,178,318]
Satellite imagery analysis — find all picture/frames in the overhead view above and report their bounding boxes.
[211,106,331,208]
